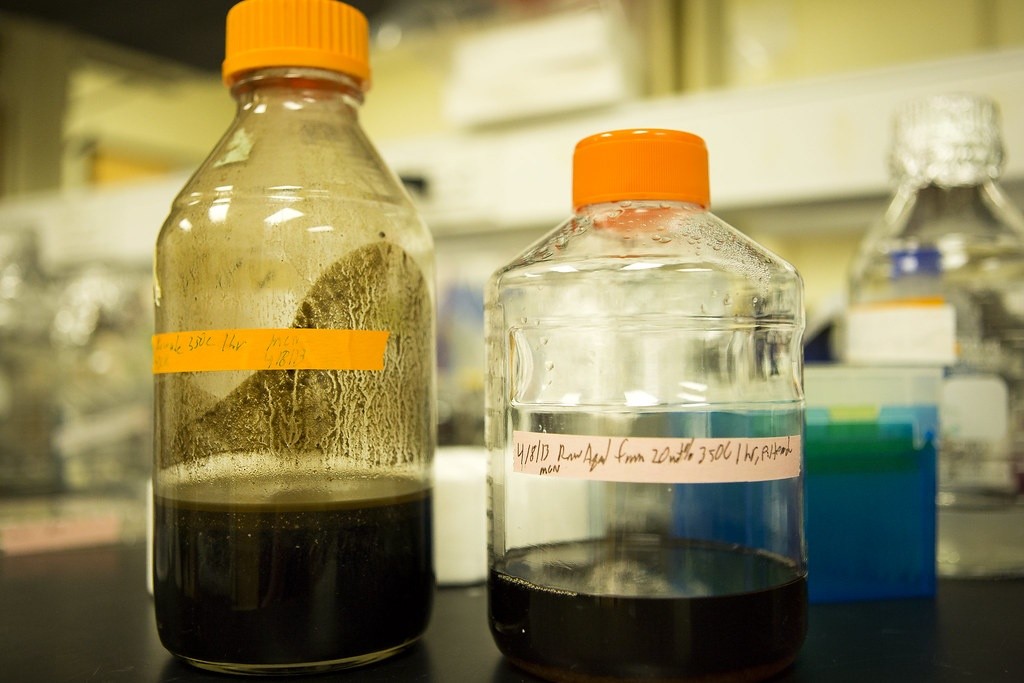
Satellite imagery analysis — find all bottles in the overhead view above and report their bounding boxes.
[832,88,1024,578]
[0,236,59,501]
[479,129,813,683]
[155,0,444,675]
